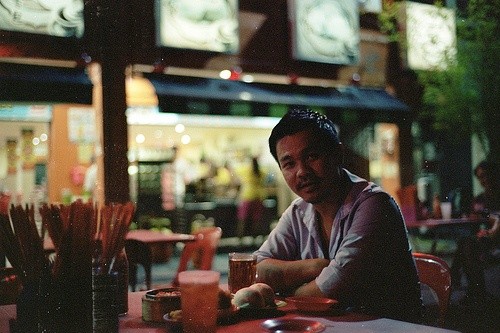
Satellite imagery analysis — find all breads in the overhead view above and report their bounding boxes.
[232,282,275,309]
[218,288,231,312]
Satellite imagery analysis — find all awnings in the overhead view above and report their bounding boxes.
[0,62,93,105]
[142,72,411,125]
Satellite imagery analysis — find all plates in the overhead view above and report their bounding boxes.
[230,300,288,313]
[259,318,327,333]
[285,296,340,315]
[164,309,181,330]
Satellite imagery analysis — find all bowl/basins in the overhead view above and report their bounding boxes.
[141,286,180,325]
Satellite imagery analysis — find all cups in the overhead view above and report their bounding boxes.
[177,270,221,333]
[16,274,119,333]
[227,252,258,298]
[439,202,452,219]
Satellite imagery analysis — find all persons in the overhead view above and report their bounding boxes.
[474,160,490,210]
[252,109,424,321]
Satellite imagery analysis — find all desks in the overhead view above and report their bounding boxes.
[406,216,489,252]
[44,231,196,292]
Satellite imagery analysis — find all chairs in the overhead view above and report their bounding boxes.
[392,187,416,226]
[412,252,452,326]
[173,226,222,283]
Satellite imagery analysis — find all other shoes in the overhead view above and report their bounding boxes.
[459,287,488,304]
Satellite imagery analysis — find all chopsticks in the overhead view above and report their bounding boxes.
[0,198,140,281]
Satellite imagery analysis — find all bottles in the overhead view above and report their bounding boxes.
[109,245,130,318]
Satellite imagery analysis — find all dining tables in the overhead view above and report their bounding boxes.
[0,286,460,333]
